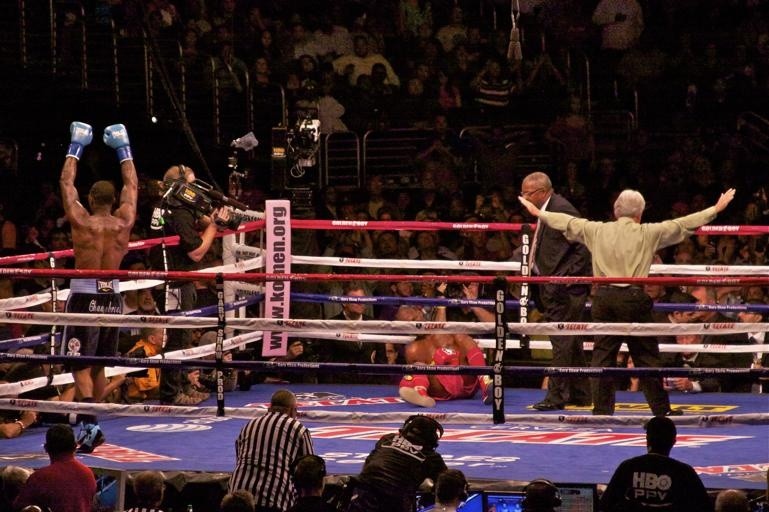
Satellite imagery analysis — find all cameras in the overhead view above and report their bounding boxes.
[163,178,249,231]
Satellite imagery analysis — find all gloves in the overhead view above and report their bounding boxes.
[102,123,134,162]
[66,121,96,160]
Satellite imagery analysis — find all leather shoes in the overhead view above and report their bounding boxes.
[531,395,562,412]
[565,396,591,406]
[656,408,684,419]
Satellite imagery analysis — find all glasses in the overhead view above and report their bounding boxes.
[521,189,540,197]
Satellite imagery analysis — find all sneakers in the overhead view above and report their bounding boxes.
[186,385,210,401]
[397,386,437,410]
[478,371,498,405]
[170,390,202,407]
[74,424,107,455]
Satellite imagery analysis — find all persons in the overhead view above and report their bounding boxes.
[126,470,167,507]
[669,189,769,269]
[290,455,330,506]
[658,334,722,395]
[232,137,514,270]
[149,166,231,405]
[417,270,448,321]
[1,465,34,506]
[716,488,751,507]
[9,423,98,511]
[689,284,755,392]
[599,416,714,511]
[515,136,593,172]
[521,172,594,411]
[60,122,137,453]
[377,270,429,363]
[595,138,769,187]
[248,307,316,385]
[122,325,167,400]
[119,1,769,136]
[430,272,496,364]
[657,291,696,345]
[342,413,447,512]
[516,189,738,420]
[0,381,36,437]
[231,390,314,509]
[735,298,769,368]
[0,134,59,380]
[428,468,467,507]
[394,303,496,408]
[318,286,393,385]
[221,487,257,506]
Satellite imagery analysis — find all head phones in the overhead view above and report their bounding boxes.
[400,415,443,448]
[431,469,467,502]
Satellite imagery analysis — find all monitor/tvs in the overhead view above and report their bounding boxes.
[416,491,483,512]
[554,483,597,512]
[483,492,527,512]
